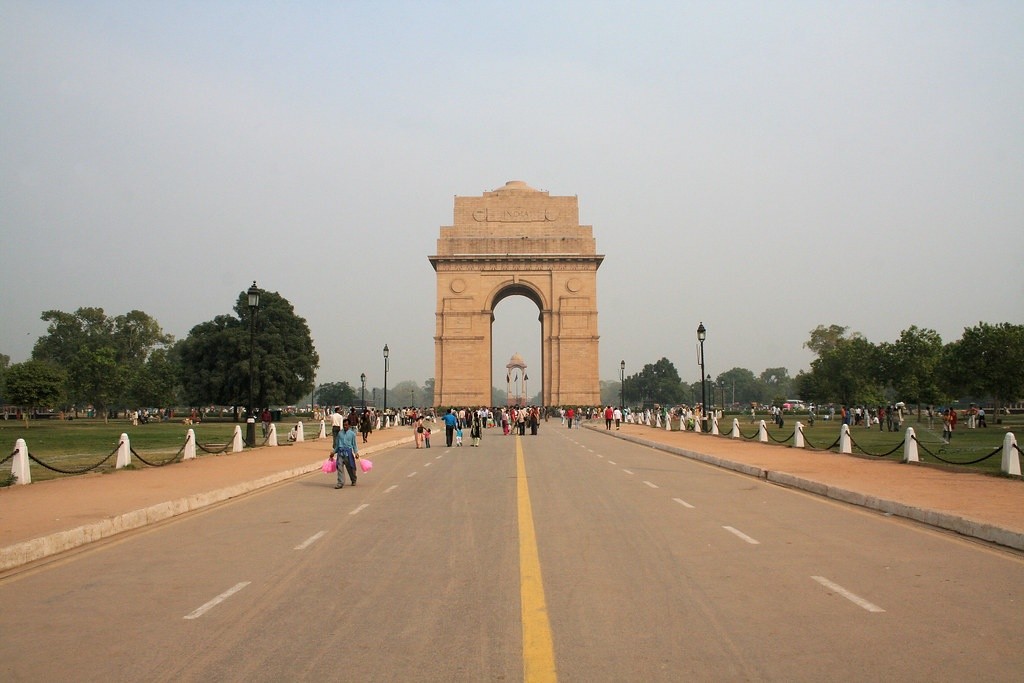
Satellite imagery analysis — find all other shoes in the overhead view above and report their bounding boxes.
[459,442,462,446]
[335,485,342,489]
[457,444,459,446]
[470,444,474,447]
[351,481,356,485]
[475,445,478,447]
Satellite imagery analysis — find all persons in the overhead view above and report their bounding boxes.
[456,426,463,447]
[561,403,703,425]
[347,408,359,436]
[470,413,482,447]
[424,429,431,448]
[414,416,428,449]
[331,407,343,449]
[4,408,28,421]
[330,419,360,489]
[368,404,438,429]
[287,425,297,441]
[125,403,333,427]
[446,403,549,436]
[360,410,372,443]
[567,407,575,429]
[261,408,271,438]
[614,408,621,430]
[943,409,953,443]
[574,409,581,429]
[605,406,613,430]
[750,401,986,433]
[59,410,64,420]
[441,409,457,447]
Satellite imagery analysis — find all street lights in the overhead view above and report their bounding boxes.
[620,360,625,422]
[695,320,710,433]
[246,279,261,450]
[382,343,390,428]
[340,386,344,407]
[659,387,662,410]
[411,390,414,407]
[360,373,367,417]
[691,383,695,406]
[707,373,711,420]
[377,394,381,410]
[721,379,725,418]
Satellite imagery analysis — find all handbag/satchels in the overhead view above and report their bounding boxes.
[418,427,423,433]
[322,458,336,473]
[358,458,372,472]
